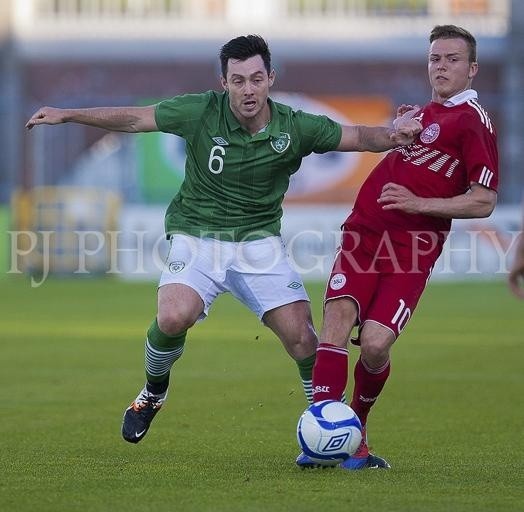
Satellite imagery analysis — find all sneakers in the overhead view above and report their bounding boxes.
[297,423,391,470]
[121,384,169,443]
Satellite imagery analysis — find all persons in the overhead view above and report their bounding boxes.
[510,232,524,297]
[24,35,423,470]
[297,26,499,470]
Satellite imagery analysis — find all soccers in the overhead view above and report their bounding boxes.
[296,400,362,465]
